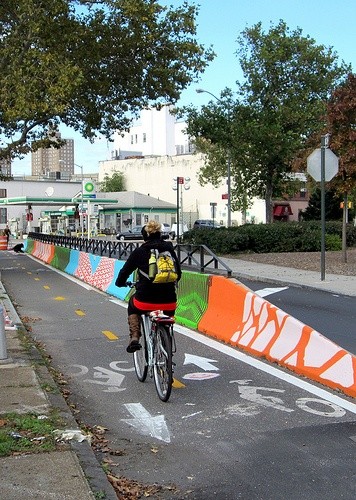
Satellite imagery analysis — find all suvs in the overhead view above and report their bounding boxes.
[194,219,225,229]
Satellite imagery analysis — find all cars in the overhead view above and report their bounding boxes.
[115,226,144,240]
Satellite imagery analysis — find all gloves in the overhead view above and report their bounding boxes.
[116,281,127,287]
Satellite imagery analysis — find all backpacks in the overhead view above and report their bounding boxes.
[139,248,178,283]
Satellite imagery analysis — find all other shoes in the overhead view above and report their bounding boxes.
[166,362,176,373]
[126,337,139,353]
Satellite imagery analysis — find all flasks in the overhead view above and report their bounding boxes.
[149,249,157,279]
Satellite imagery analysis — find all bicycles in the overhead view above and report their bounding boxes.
[120,281,177,402]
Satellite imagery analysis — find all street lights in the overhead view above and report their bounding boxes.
[172,176,191,238]
[59,159,84,238]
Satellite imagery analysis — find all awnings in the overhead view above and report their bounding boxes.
[274,205,293,216]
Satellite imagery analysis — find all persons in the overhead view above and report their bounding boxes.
[2,225,11,247]
[13,243,24,253]
[115,220,182,384]
